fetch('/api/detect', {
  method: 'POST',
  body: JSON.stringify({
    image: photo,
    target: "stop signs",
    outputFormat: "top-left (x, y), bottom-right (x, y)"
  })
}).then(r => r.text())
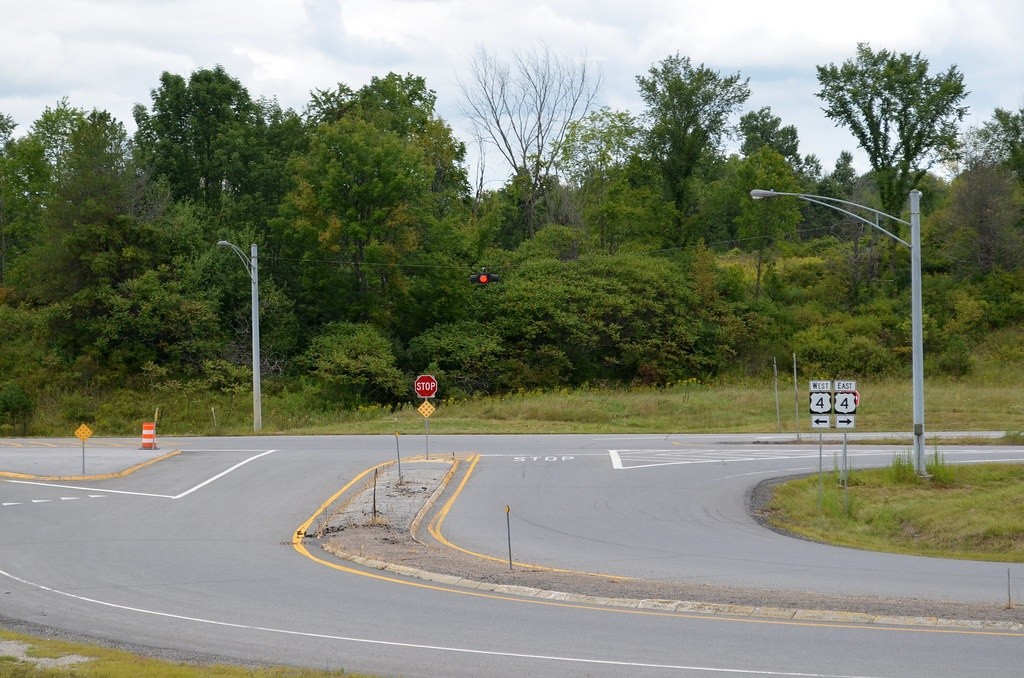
top-left (414, 375), bottom-right (437, 398)
top-left (840, 390), bottom-right (860, 407)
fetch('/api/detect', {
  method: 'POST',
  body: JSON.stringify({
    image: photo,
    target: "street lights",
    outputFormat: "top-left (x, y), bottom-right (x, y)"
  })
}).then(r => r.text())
top-left (218, 241), bottom-right (261, 436)
top-left (749, 189), bottom-right (926, 479)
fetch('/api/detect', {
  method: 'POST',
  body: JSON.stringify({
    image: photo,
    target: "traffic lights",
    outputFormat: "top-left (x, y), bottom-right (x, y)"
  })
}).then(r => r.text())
top-left (471, 272), bottom-right (500, 284)
